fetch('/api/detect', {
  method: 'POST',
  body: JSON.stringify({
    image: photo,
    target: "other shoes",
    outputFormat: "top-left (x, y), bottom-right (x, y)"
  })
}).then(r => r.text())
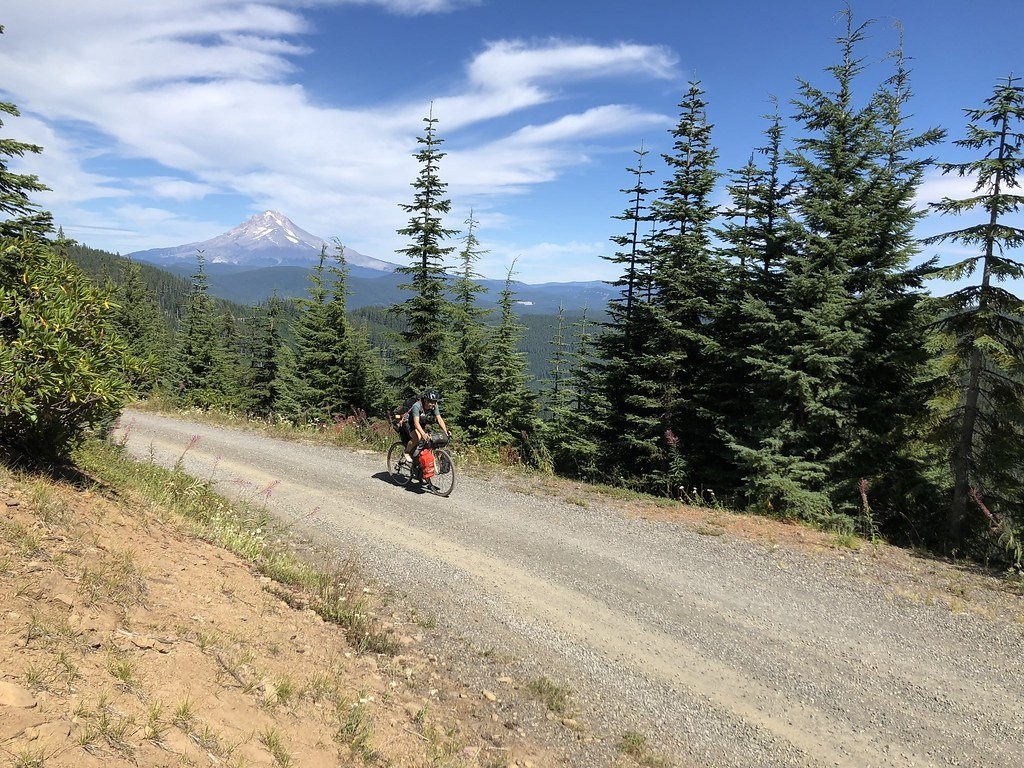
top-left (402, 450), bottom-right (413, 463)
top-left (412, 473), bottom-right (428, 484)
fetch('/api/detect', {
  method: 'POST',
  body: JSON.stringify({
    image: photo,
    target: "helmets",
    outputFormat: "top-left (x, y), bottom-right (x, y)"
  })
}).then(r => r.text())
top-left (424, 389), bottom-right (439, 401)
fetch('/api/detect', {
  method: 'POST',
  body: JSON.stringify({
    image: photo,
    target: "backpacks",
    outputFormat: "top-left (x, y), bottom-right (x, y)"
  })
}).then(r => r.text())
top-left (392, 394), bottom-right (423, 433)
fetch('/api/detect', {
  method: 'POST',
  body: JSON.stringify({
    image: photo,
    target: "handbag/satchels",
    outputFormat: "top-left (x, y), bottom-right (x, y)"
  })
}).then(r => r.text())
top-left (438, 449), bottom-right (450, 473)
top-left (419, 449), bottom-right (435, 479)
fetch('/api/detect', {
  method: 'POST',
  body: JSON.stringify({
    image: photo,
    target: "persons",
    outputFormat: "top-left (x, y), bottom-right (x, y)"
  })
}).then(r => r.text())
top-left (399, 389), bottom-right (451, 485)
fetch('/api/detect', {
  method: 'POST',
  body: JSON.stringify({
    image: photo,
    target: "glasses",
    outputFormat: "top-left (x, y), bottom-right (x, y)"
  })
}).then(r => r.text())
top-left (427, 399), bottom-right (436, 404)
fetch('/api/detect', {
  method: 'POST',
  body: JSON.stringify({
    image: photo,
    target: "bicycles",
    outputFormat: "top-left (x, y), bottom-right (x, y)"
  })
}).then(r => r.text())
top-left (386, 425), bottom-right (456, 498)
